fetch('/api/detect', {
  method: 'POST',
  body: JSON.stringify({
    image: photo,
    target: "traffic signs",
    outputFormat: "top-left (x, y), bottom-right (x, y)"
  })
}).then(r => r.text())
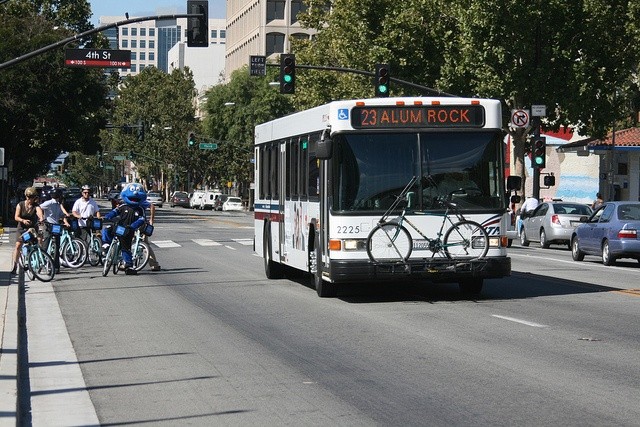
top-left (511, 109), bottom-right (529, 127)
top-left (250, 55), bottom-right (267, 75)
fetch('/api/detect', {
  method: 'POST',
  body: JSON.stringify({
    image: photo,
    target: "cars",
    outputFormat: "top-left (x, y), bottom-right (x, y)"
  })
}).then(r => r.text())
top-left (222, 196), bottom-right (244, 211)
top-left (64, 189), bottom-right (92, 212)
top-left (214, 194), bottom-right (235, 210)
top-left (520, 202), bottom-right (599, 248)
top-left (571, 201), bottom-right (639, 265)
top-left (145, 192), bottom-right (163, 207)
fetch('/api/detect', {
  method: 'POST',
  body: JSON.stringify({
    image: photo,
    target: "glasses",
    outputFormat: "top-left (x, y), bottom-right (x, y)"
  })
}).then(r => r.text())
top-left (27, 195), bottom-right (35, 198)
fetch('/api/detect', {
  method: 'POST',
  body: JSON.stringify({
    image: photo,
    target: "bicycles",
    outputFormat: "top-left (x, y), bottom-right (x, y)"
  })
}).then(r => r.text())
top-left (130, 218), bottom-right (154, 271)
top-left (41, 224), bottom-right (88, 268)
top-left (65, 216), bottom-right (102, 265)
top-left (18, 221), bottom-right (54, 281)
top-left (366, 192), bottom-right (489, 268)
top-left (102, 218), bottom-right (137, 276)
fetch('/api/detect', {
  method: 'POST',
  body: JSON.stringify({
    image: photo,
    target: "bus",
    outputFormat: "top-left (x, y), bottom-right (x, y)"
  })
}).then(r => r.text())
top-left (253, 96), bottom-right (510, 297)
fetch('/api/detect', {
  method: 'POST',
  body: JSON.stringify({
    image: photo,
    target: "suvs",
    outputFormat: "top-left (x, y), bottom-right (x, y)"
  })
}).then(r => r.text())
top-left (188, 191), bottom-right (206, 208)
top-left (171, 193), bottom-right (188, 208)
top-left (200, 191), bottom-right (222, 209)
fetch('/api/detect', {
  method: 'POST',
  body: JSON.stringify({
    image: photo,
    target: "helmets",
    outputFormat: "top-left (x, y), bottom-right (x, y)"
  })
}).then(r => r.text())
top-left (51, 189), bottom-right (62, 198)
top-left (82, 184), bottom-right (90, 189)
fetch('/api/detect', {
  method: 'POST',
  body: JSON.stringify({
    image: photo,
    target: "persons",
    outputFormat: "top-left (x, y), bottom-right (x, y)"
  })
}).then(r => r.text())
top-left (63, 217), bottom-right (70, 227)
top-left (10, 186), bottom-right (43, 275)
top-left (591, 192), bottom-right (604, 210)
top-left (39, 187), bottom-right (63, 274)
top-left (521, 195), bottom-right (539, 215)
top-left (143, 200), bottom-right (161, 272)
top-left (72, 184), bottom-right (103, 254)
top-left (111, 198), bottom-right (123, 210)
top-left (597, 199), bottom-right (604, 209)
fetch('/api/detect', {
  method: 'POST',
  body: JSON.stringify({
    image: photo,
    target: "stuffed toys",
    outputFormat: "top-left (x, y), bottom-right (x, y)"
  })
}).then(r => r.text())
top-left (99, 182), bottom-right (147, 275)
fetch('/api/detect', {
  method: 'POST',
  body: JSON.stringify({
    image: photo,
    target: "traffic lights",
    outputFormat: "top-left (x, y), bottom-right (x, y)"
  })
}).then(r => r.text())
top-left (137, 120), bottom-right (144, 141)
top-left (127, 123), bottom-right (132, 134)
top-left (187, 0), bottom-right (209, 47)
top-left (544, 175), bottom-right (555, 186)
top-left (129, 150), bottom-right (133, 159)
top-left (531, 137), bottom-right (545, 167)
top-left (121, 123), bottom-right (127, 133)
top-left (375, 64), bottom-right (389, 97)
top-left (280, 54), bottom-right (295, 93)
top-left (188, 132), bottom-right (195, 150)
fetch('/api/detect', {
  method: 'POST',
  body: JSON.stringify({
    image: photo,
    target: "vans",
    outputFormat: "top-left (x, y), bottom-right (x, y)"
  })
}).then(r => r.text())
top-left (171, 190), bottom-right (188, 201)
